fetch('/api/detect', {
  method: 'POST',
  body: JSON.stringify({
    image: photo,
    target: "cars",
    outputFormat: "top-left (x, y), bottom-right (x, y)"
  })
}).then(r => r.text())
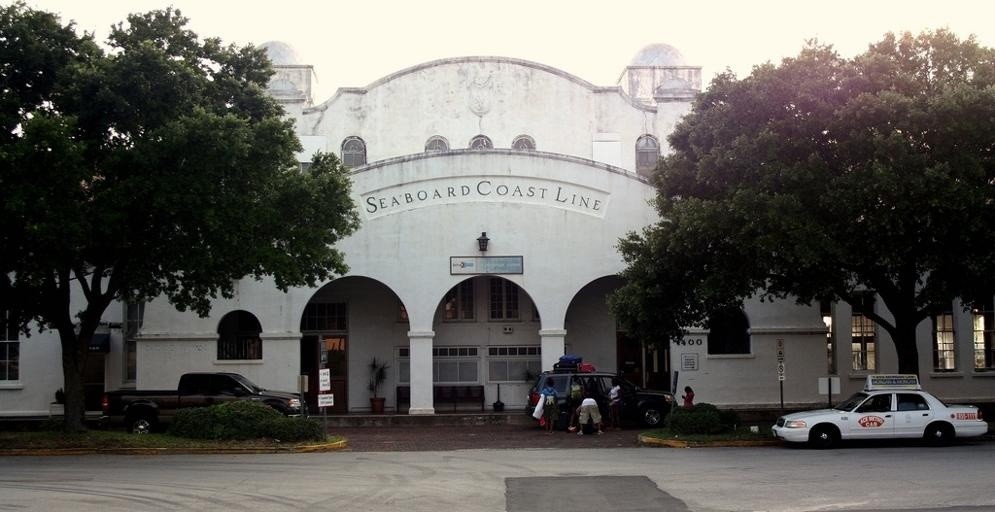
top-left (770, 374), bottom-right (989, 446)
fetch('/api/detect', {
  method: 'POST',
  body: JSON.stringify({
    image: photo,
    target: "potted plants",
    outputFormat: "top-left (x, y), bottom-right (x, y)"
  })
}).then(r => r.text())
top-left (367, 356), bottom-right (391, 412)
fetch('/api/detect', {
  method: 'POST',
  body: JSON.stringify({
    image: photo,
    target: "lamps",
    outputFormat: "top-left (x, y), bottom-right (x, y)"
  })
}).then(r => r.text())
top-left (476, 232), bottom-right (490, 251)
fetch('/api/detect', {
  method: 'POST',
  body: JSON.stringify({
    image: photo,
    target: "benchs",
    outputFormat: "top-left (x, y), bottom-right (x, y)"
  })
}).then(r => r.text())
top-left (396, 385), bottom-right (485, 413)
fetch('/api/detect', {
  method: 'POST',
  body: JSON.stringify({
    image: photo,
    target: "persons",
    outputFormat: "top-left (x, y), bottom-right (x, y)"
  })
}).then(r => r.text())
top-left (539, 377), bottom-right (561, 438)
top-left (569, 375), bottom-right (583, 427)
top-left (576, 397), bottom-right (605, 436)
top-left (682, 385), bottom-right (695, 410)
top-left (607, 378), bottom-right (623, 432)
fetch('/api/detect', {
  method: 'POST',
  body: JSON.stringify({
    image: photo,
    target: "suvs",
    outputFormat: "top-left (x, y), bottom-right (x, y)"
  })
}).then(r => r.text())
top-left (524, 363), bottom-right (680, 429)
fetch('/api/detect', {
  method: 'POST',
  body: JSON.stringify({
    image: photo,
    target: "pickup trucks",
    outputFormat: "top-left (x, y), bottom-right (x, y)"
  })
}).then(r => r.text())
top-left (99, 370), bottom-right (310, 436)
